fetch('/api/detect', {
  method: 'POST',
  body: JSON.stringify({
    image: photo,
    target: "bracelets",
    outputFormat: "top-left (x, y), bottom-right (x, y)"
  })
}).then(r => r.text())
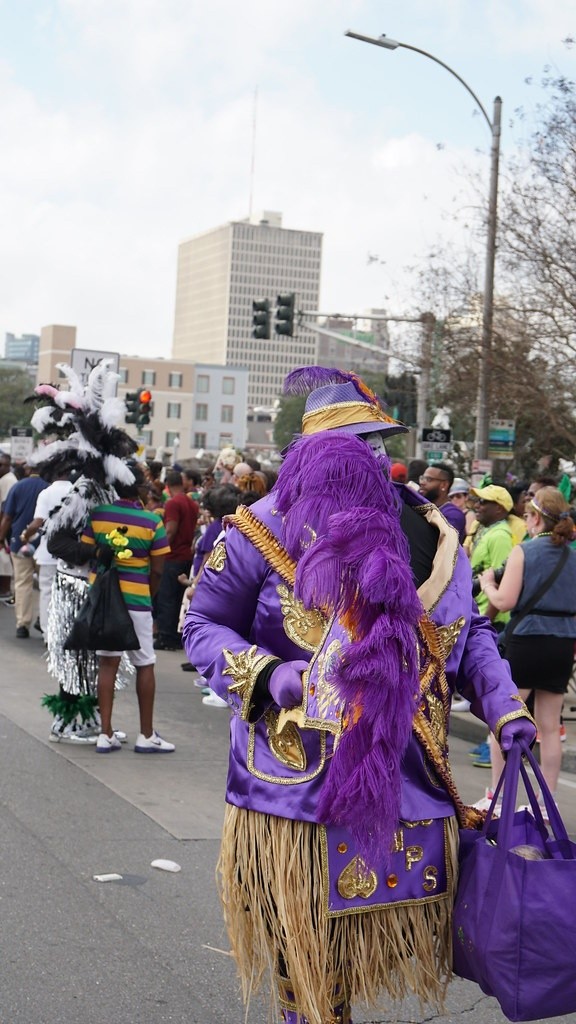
top-left (21, 534), bottom-right (26, 541)
top-left (482, 584), bottom-right (492, 593)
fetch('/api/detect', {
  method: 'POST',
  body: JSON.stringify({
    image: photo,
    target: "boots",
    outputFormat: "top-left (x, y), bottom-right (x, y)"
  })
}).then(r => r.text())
top-left (277, 972), bottom-right (353, 1024)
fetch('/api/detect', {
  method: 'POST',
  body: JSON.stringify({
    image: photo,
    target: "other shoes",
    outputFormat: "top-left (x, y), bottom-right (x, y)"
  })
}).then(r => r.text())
top-left (42, 694), bottom-right (98, 745)
top-left (16, 626), bottom-right (29, 637)
top-left (33, 616), bottom-right (43, 633)
top-left (153, 632), bottom-right (228, 707)
top-left (80, 697), bottom-right (127, 741)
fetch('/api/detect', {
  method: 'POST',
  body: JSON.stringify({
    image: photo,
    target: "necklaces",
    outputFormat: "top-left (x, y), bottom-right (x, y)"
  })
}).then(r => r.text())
top-left (537, 532), bottom-right (553, 537)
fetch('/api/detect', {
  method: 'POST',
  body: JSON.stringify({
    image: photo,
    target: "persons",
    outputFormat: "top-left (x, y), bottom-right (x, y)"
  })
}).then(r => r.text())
top-left (391, 455), bottom-right (570, 714)
top-left (44, 451), bottom-right (127, 744)
top-left (472, 487), bottom-right (576, 823)
top-left (0, 454), bottom-right (74, 645)
top-left (182, 366), bottom-right (539, 1024)
top-left (147, 449), bottom-right (278, 709)
top-left (91, 462), bottom-right (175, 753)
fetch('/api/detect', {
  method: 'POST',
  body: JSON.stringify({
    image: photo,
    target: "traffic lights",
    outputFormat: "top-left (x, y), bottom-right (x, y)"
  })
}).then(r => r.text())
top-left (381, 373), bottom-right (417, 428)
top-left (135, 388), bottom-right (152, 425)
top-left (273, 292), bottom-right (296, 337)
top-left (251, 296), bottom-right (272, 340)
top-left (124, 391), bottom-right (137, 424)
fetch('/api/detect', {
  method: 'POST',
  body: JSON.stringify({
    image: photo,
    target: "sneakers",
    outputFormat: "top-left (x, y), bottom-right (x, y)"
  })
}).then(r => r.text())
top-left (97, 728), bottom-right (122, 753)
top-left (450, 693), bottom-right (566, 822)
top-left (134, 729), bottom-right (175, 753)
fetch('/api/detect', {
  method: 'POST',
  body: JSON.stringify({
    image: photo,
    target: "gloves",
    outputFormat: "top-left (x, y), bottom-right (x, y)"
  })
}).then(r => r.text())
top-left (498, 715), bottom-right (537, 762)
top-left (268, 660), bottom-right (309, 710)
top-left (95, 544), bottom-right (115, 569)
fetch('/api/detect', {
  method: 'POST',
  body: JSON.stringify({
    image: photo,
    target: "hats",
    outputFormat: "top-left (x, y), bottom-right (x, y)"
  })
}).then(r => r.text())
top-left (448, 478), bottom-right (471, 497)
top-left (391, 463), bottom-right (406, 480)
top-left (280, 366), bottom-right (409, 460)
top-left (473, 484), bottom-right (514, 512)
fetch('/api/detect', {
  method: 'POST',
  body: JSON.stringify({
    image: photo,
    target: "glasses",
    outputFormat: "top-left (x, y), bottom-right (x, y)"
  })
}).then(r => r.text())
top-left (524, 491), bottom-right (535, 498)
top-left (419, 476), bottom-right (446, 482)
top-left (450, 493), bottom-right (462, 499)
top-left (473, 497), bottom-right (498, 505)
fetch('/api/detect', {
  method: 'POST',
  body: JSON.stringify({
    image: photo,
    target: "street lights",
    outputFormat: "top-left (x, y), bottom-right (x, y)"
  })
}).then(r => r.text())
top-left (171, 437), bottom-right (181, 467)
top-left (343, 27), bottom-right (505, 489)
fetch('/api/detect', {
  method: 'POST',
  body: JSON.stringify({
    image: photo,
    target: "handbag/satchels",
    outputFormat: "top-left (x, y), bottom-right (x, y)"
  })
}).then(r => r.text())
top-left (446, 738), bottom-right (576, 1021)
top-left (62, 559), bottom-right (141, 652)
